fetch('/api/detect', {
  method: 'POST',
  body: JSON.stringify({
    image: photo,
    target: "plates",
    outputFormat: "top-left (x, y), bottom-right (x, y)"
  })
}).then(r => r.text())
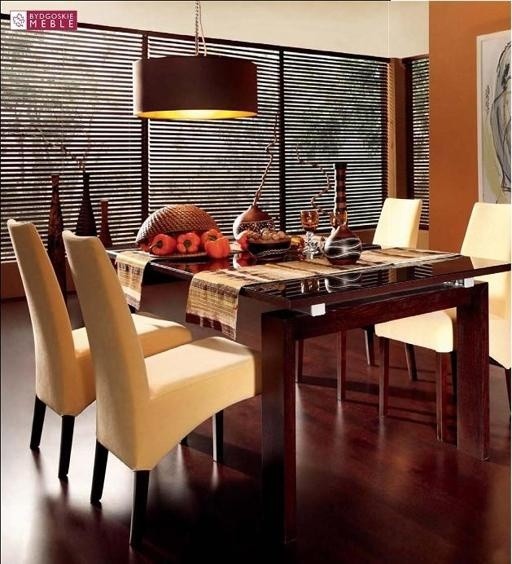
top-left (148, 251), bottom-right (207, 260)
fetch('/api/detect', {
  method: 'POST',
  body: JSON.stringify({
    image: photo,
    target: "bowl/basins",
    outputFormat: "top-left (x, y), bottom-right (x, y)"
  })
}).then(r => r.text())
top-left (246, 237), bottom-right (291, 261)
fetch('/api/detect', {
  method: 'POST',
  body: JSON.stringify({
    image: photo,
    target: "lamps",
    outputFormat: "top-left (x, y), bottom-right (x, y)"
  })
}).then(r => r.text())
top-left (132, 3), bottom-right (259, 122)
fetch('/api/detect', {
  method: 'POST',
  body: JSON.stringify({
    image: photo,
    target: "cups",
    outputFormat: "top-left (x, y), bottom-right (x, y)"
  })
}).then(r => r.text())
top-left (329, 210), bottom-right (348, 230)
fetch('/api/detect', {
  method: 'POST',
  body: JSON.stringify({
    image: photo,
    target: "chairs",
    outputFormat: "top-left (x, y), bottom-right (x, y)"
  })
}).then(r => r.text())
top-left (362, 195), bottom-right (512, 430)
top-left (5, 219), bottom-right (261, 546)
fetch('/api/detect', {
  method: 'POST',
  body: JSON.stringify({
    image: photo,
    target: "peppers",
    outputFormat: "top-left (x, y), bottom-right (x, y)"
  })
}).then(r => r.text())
top-left (205, 236), bottom-right (230, 259)
top-left (177, 232), bottom-right (201, 254)
top-left (149, 234), bottom-right (176, 256)
top-left (200, 229), bottom-right (222, 250)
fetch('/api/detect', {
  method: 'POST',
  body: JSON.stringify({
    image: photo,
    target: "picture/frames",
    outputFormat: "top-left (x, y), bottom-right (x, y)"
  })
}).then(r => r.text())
top-left (475, 28), bottom-right (511, 203)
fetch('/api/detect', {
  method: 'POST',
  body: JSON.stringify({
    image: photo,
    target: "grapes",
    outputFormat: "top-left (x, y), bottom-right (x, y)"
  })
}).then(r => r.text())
top-left (247, 228), bottom-right (287, 243)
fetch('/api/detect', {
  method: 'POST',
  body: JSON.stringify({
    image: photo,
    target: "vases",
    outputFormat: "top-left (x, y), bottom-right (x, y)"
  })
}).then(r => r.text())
top-left (324, 226), bottom-right (362, 266)
top-left (232, 206), bottom-right (274, 244)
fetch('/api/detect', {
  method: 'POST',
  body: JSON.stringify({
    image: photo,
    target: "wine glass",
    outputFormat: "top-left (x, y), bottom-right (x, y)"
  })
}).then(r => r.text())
top-left (300, 210), bottom-right (320, 254)
